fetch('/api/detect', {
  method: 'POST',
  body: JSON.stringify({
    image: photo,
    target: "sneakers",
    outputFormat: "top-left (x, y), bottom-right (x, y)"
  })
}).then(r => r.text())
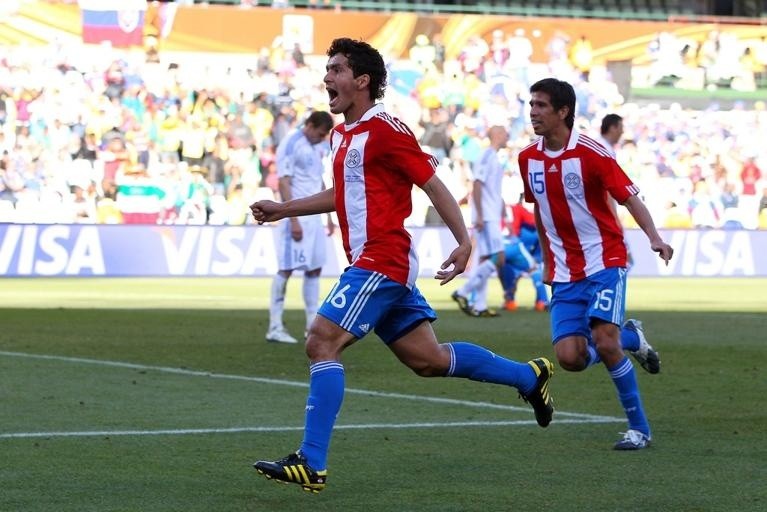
top-left (252, 450), bottom-right (327, 494)
top-left (452, 291), bottom-right (501, 318)
top-left (265, 327), bottom-right (298, 344)
top-left (622, 319), bottom-right (661, 375)
top-left (504, 300), bottom-right (545, 311)
top-left (518, 357), bottom-right (555, 428)
top-left (614, 429), bottom-right (653, 451)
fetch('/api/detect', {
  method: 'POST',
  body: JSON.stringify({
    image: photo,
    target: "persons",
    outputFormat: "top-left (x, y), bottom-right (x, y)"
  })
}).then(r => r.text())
top-left (514, 75), bottom-right (676, 452)
top-left (516, 191), bottom-right (554, 308)
top-left (446, 122), bottom-right (514, 322)
top-left (247, 33), bottom-right (557, 497)
top-left (0, 28), bottom-right (767, 231)
top-left (263, 108), bottom-right (336, 346)
top-left (491, 198), bottom-right (549, 314)
